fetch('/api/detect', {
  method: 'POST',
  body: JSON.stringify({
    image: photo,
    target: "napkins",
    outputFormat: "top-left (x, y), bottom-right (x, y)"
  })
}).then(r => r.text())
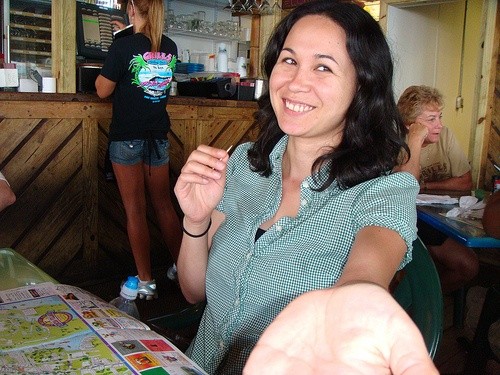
top-left (416, 194), bottom-right (459, 205)
top-left (445, 196), bottom-right (478, 218)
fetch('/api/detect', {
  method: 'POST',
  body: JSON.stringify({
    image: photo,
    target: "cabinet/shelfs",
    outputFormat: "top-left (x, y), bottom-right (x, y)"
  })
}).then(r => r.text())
top-left (0, 0), bottom-right (76, 93)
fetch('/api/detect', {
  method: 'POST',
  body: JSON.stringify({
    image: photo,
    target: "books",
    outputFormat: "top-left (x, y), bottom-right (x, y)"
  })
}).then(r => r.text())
top-left (110, 23), bottom-right (134, 39)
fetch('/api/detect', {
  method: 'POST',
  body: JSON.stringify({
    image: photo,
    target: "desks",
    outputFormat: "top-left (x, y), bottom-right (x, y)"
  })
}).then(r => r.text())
top-left (416, 188), bottom-right (500, 375)
top-left (0, 246), bottom-right (62, 292)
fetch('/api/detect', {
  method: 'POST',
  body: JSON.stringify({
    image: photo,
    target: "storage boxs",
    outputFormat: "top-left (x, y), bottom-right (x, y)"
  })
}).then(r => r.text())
top-left (176, 74), bottom-right (269, 101)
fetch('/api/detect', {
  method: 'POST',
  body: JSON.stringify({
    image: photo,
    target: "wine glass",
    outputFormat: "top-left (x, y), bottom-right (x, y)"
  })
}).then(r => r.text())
top-left (224, 0), bottom-right (286, 15)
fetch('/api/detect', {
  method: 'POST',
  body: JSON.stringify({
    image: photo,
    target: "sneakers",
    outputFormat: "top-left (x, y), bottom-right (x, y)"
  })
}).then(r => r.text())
top-left (119, 275), bottom-right (159, 300)
top-left (166, 263), bottom-right (179, 280)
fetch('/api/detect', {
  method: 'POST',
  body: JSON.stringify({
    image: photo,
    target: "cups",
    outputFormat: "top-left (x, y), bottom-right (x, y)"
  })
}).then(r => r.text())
top-left (236, 56), bottom-right (247, 79)
top-left (218, 43), bottom-right (227, 72)
top-left (163, 9), bottom-right (245, 42)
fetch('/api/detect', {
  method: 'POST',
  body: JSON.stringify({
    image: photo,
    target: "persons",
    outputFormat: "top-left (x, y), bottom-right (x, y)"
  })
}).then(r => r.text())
top-left (173, 0), bottom-right (441, 374)
top-left (457, 189), bottom-right (500, 375)
top-left (389, 86), bottom-right (480, 298)
top-left (0, 171), bottom-right (17, 212)
top-left (94, 0), bottom-right (182, 302)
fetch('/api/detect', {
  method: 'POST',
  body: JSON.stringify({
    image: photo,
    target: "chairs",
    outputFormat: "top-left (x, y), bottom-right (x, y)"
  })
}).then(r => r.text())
top-left (140, 234), bottom-right (445, 362)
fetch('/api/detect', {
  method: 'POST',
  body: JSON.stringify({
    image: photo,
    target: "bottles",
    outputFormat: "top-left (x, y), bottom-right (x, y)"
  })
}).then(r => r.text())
top-left (109, 277), bottom-right (140, 320)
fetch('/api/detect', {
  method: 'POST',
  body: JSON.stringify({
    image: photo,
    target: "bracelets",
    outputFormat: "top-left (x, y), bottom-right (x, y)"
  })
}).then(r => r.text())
top-left (182, 218), bottom-right (213, 238)
top-left (424, 183), bottom-right (427, 192)
